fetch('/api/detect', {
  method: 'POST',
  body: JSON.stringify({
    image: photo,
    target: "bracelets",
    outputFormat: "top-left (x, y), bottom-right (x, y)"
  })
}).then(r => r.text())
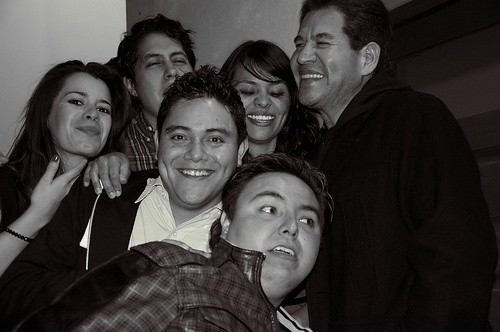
top-left (3, 228), bottom-right (35, 243)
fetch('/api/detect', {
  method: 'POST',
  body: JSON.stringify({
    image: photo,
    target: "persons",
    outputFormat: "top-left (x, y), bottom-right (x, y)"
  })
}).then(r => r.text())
top-left (104, 56), bottom-right (117, 72)
top-left (14, 152), bottom-right (312, 332)
top-left (75, 63), bottom-right (249, 274)
top-left (0, 61), bottom-right (132, 332)
top-left (289, 0), bottom-right (497, 332)
top-left (220, 39), bottom-right (299, 162)
top-left (109, 12), bottom-right (199, 171)
